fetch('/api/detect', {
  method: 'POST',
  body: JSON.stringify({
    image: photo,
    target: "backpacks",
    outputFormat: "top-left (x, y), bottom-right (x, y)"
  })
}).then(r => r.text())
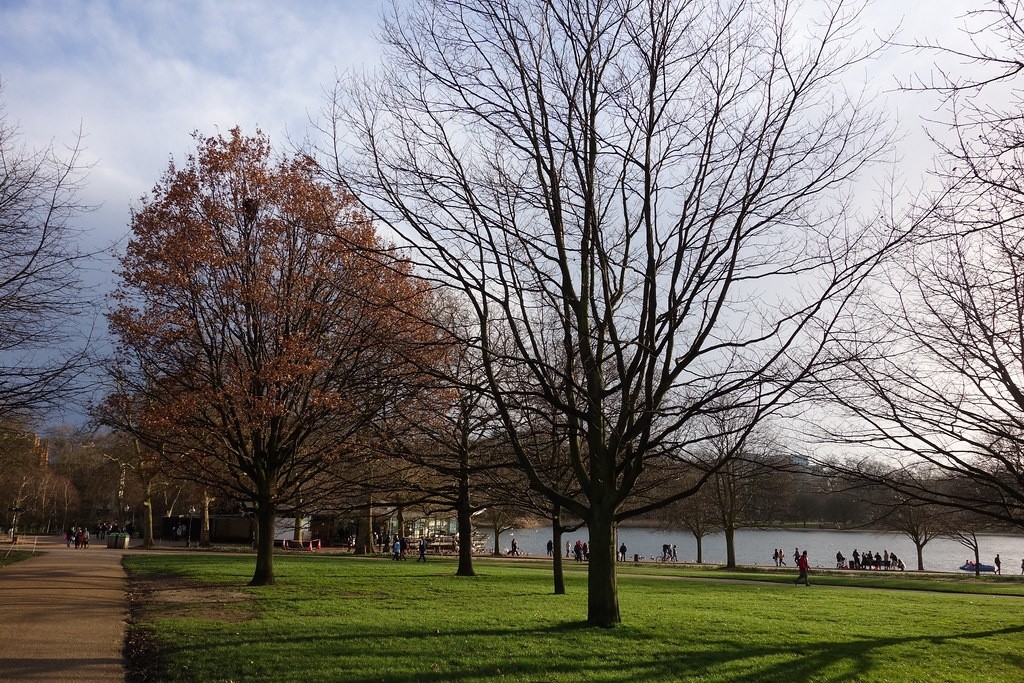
top-left (995, 557), bottom-right (997, 563)
top-left (663, 545), bottom-right (668, 547)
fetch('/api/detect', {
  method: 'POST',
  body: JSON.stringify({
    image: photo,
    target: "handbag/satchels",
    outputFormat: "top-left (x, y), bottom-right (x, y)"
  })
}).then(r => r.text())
top-left (799, 555), bottom-right (800, 557)
top-left (64, 535), bottom-right (68, 541)
top-left (779, 565), bottom-right (781, 566)
top-left (773, 556), bottom-right (775, 559)
top-left (86, 540), bottom-right (89, 544)
top-left (779, 554), bottom-right (784, 558)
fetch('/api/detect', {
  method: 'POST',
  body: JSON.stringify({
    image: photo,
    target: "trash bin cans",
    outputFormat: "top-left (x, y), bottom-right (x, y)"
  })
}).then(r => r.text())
top-left (634, 553), bottom-right (641, 563)
top-left (116, 533), bottom-right (130, 549)
top-left (849, 561), bottom-right (854, 570)
top-left (107, 532), bottom-right (120, 549)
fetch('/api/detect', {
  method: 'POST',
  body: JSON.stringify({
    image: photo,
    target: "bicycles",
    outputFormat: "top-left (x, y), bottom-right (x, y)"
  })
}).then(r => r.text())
top-left (656, 551), bottom-right (676, 564)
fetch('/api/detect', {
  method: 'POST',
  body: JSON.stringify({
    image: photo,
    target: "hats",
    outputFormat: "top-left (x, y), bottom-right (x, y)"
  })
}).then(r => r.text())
top-left (1022, 559), bottom-right (1024, 561)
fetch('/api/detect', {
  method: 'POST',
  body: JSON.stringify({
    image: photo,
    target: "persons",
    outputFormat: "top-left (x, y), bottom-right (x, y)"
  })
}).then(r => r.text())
top-left (346, 531), bottom-right (629, 564)
top-left (965, 554), bottom-right (1024, 577)
top-left (834, 549), bottom-right (907, 573)
top-left (7, 519), bottom-right (190, 549)
top-left (773, 547), bottom-right (802, 566)
top-left (662, 543), bottom-right (677, 562)
top-left (793, 550), bottom-right (812, 589)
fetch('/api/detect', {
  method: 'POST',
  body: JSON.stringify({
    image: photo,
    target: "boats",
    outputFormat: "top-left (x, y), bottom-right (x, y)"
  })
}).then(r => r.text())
top-left (960, 564), bottom-right (996, 572)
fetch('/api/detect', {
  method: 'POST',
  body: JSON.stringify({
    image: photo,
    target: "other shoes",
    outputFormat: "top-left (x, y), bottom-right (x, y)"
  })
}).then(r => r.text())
top-left (806, 584), bottom-right (811, 586)
top-left (794, 580), bottom-right (797, 585)
top-left (777, 565), bottom-right (778, 566)
top-left (785, 564), bottom-right (786, 566)
top-left (994, 571), bottom-right (996, 574)
top-left (796, 565), bottom-right (799, 567)
top-left (999, 574), bottom-right (1001, 575)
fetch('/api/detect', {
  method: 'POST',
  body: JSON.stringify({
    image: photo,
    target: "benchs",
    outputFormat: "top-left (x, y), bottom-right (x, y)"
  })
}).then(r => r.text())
top-left (860, 560), bottom-right (891, 570)
